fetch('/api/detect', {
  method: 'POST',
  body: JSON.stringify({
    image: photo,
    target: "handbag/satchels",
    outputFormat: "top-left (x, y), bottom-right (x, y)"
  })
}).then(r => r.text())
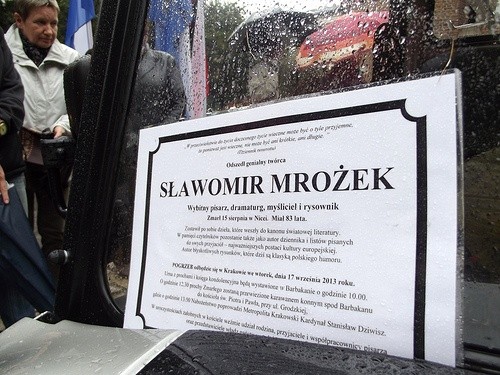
top-left (40, 138), bottom-right (75, 167)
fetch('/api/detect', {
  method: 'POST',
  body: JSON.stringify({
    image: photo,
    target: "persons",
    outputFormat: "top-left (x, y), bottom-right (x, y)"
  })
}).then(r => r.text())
top-left (0, 0), bottom-right (185, 328)
top-left (239, 40), bottom-right (365, 100)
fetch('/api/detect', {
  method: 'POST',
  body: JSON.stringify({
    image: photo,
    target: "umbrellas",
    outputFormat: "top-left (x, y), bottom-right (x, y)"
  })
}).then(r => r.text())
top-left (226, 2), bottom-right (387, 69)
